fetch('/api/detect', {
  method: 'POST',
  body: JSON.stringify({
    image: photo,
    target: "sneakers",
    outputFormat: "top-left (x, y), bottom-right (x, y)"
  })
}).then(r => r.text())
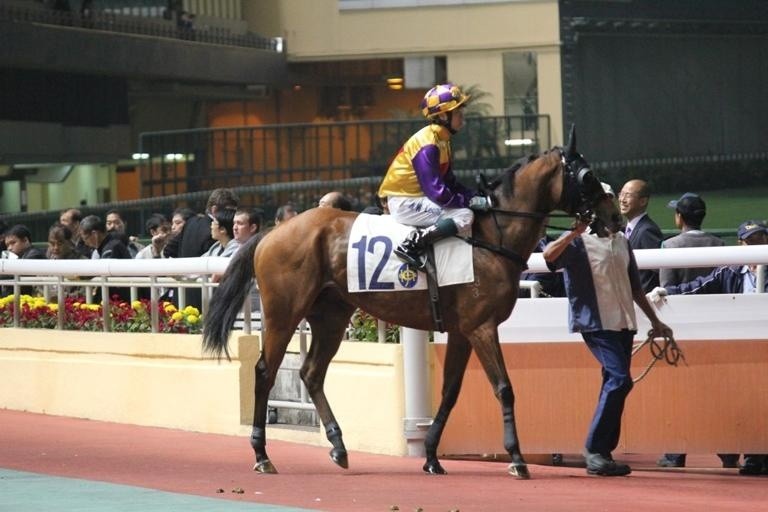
top-left (719, 460), bottom-right (741, 469)
top-left (654, 455), bottom-right (686, 468)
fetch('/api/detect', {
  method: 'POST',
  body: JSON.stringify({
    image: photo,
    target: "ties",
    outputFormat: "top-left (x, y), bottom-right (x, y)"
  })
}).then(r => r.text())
top-left (622, 226), bottom-right (632, 241)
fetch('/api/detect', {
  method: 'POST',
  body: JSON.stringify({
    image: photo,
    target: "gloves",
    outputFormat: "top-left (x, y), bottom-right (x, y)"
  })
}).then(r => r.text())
top-left (646, 286), bottom-right (669, 304)
top-left (467, 194), bottom-right (498, 212)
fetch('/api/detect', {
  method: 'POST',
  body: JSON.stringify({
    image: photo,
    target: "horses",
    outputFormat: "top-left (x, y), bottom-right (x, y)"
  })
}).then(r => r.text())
top-left (202, 122), bottom-right (623, 481)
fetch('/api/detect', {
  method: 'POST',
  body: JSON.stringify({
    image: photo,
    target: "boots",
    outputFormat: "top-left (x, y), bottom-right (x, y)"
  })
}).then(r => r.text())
top-left (391, 224), bottom-right (443, 274)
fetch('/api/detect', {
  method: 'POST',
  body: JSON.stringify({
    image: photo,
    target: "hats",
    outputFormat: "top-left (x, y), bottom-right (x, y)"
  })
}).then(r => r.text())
top-left (737, 219), bottom-right (768, 242)
top-left (600, 181), bottom-right (615, 198)
top-left (665, 193), bottom-right (707, 219)
top-left (420, 80), bottom-right (471, 120)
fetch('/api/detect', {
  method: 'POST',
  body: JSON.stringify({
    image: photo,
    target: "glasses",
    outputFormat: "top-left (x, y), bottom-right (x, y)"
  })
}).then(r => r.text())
top-left (617, 192), bottom-right (640, 201)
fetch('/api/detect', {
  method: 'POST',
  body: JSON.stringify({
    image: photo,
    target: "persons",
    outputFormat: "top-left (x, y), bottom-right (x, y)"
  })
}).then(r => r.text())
top-left (518, 217), bottom-right (565, 297)
top-left (646, 220), bottom-right (768, 474)
top-left (48, 224), bottom-right (77, 259)
top-left (200, 208), bottom-right (239, 282)
top-left (163, 187), bottom-right (240, 312)
top-left (655, 193), bottom-right (740, 468)
top-left (543, 181), bottom-right (664, 476)
top-left (618, 179), bottom-right (662, 294)
top-left (378, 84), bottom-right (488, 273)
top-left (135, 214), bottom-right (172, 298)
top-left (5, 224), bottom-right (46, 259)
top-left (375, 190), bottom-right (390, 214)
top-left (152, 208), bottom-right (196, 302)
top-left (318, 191), bottom-right (352, 212)
top-left (274, 204), bottom-right (297, 225)
top-left (80, 215), bottom-right (132, 258)
top-left (61, 209), bottom-right (89, 256)
top-left (232, 208), bottom-right (260, 258)
top-left (91, 208), bottom-right (136, 258)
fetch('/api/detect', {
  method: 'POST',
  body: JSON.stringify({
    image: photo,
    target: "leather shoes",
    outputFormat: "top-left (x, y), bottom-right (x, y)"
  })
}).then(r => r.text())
top-left (582, 452), bottom-right (615, 476)
top-left (605, 455), bottom-right (631, 477)
